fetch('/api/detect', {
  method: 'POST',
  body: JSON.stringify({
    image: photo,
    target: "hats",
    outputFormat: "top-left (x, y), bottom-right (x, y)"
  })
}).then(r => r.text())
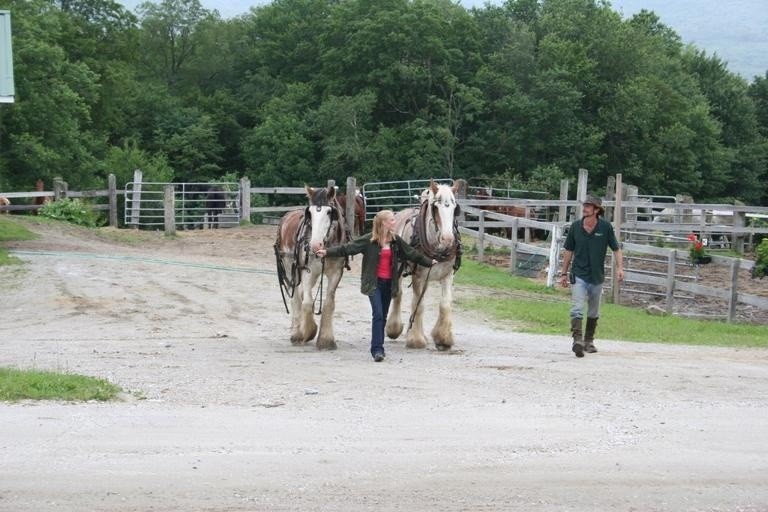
top-left (579, 194), bottom-right (606, 218)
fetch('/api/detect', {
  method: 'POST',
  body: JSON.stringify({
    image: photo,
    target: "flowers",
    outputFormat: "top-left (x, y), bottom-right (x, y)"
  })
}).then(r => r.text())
top-left (684, 235), bottom-right (705, 265)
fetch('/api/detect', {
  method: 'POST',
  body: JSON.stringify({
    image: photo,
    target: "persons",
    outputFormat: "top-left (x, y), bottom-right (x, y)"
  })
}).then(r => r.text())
top-left (318, 210), bottom-right (438, 362)
top-left (560, 194), bottom-right (625, 357)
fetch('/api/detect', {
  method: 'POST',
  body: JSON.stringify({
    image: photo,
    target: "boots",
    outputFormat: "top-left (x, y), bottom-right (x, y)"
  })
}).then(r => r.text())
top-left (583, 316), bottom-right (600, 353)
top-left (571, 317), bottom-right (583, 357)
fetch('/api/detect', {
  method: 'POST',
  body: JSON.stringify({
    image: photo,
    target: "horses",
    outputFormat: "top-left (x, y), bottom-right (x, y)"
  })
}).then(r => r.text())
top-left (190, 180), bottom-right (225, 229)
top-left (274, 181), bottom-right (351, 352)
top-left (473, 184), bottom-right (537, 244)
top-left (0, 193), bottom-right (14, 215)
top-left (338, 193), bottom-right (366, 236)
top-left (384, 176), bottom-right (468, 351)
top-left (31, 176), bottom-right (51, 214)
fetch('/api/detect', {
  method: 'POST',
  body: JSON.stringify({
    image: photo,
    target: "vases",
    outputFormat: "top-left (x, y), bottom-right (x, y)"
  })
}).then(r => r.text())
top-left (693, 255), bottom-right (712, 265)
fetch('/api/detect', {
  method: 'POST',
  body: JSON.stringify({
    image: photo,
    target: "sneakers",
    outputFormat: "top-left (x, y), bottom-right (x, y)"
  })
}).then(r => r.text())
top-left (373, 352), bottom-right (385, 361)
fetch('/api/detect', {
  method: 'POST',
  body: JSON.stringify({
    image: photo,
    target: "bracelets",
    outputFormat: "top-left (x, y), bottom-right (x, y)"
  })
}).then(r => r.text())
top-left (562, 273), bottom-right (568, 276)
